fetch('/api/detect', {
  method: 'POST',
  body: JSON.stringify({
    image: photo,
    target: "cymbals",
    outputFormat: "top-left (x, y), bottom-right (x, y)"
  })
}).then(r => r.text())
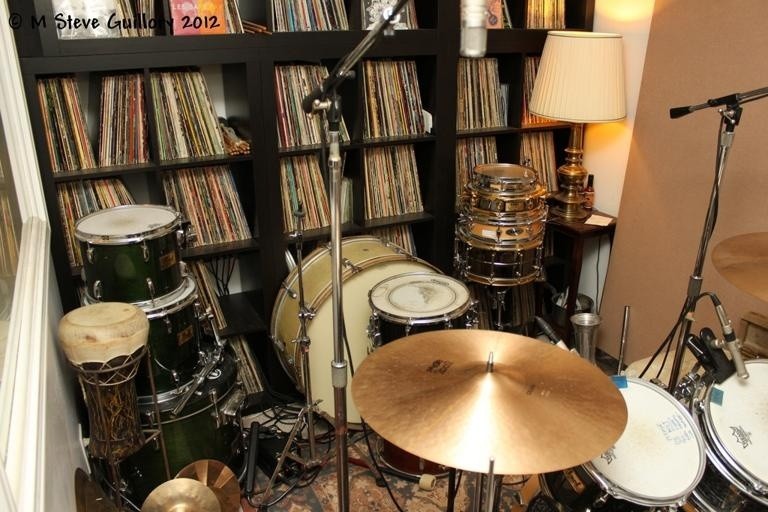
top-left (140, 478), bottom-right (222, 512)
top-left (352, 330), bottom-right (628, 477)
top-left (712, 231), bottom-right (768, 303)
top-left (75, 467), bottom-right (119, 512)
top-left (173, 460), bottom-right (242, 512)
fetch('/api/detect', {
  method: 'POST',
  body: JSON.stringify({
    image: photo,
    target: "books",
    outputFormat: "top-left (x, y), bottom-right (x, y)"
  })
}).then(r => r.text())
top-left (489, 1), bottom-right (567, 32)
top-left (114, 0), bottom-right (354, 38)
top-left (34, 60), bottom-right (566, 395)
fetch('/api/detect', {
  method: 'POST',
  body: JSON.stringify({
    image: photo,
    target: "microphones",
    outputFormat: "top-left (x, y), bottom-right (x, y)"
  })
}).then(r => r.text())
top-left (704, 292), bottom-right (750, 382)
top-left (457, 0), bottom-right (490, 61)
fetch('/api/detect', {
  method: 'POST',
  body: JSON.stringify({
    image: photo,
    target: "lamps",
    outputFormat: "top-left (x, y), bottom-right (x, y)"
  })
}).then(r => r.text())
top-left (527, 26), bottom-right (627, 222)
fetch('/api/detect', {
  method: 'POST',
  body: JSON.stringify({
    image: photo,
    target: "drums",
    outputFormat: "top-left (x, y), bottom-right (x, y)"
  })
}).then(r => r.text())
top-left (691, 358), bottom-right (767, 511)
top-left (364, 272), bottom-right (481, 481)
top-left (92, 348), bottom-right (249, 512)
top-left (73, 205), bottom-right (199, 309)
top-left (539, 376), bottom-right (708, 509)
top-left (267, 234), bottom-right (446, 432)
top-left (452, 163), bottom-right (549, 288)
top-left (58, 301), bottom-right (151, 463)
top-left (80, 275), bottom-right (212, 404)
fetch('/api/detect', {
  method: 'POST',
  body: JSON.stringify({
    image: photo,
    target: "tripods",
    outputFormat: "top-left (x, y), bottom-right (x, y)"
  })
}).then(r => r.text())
top-left (256, 232), bottom-right (386, 512)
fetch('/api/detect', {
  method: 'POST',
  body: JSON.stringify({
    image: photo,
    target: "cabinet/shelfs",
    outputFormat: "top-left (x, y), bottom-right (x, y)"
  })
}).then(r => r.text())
top-left (11, 0), bottom-right (596, 417)
